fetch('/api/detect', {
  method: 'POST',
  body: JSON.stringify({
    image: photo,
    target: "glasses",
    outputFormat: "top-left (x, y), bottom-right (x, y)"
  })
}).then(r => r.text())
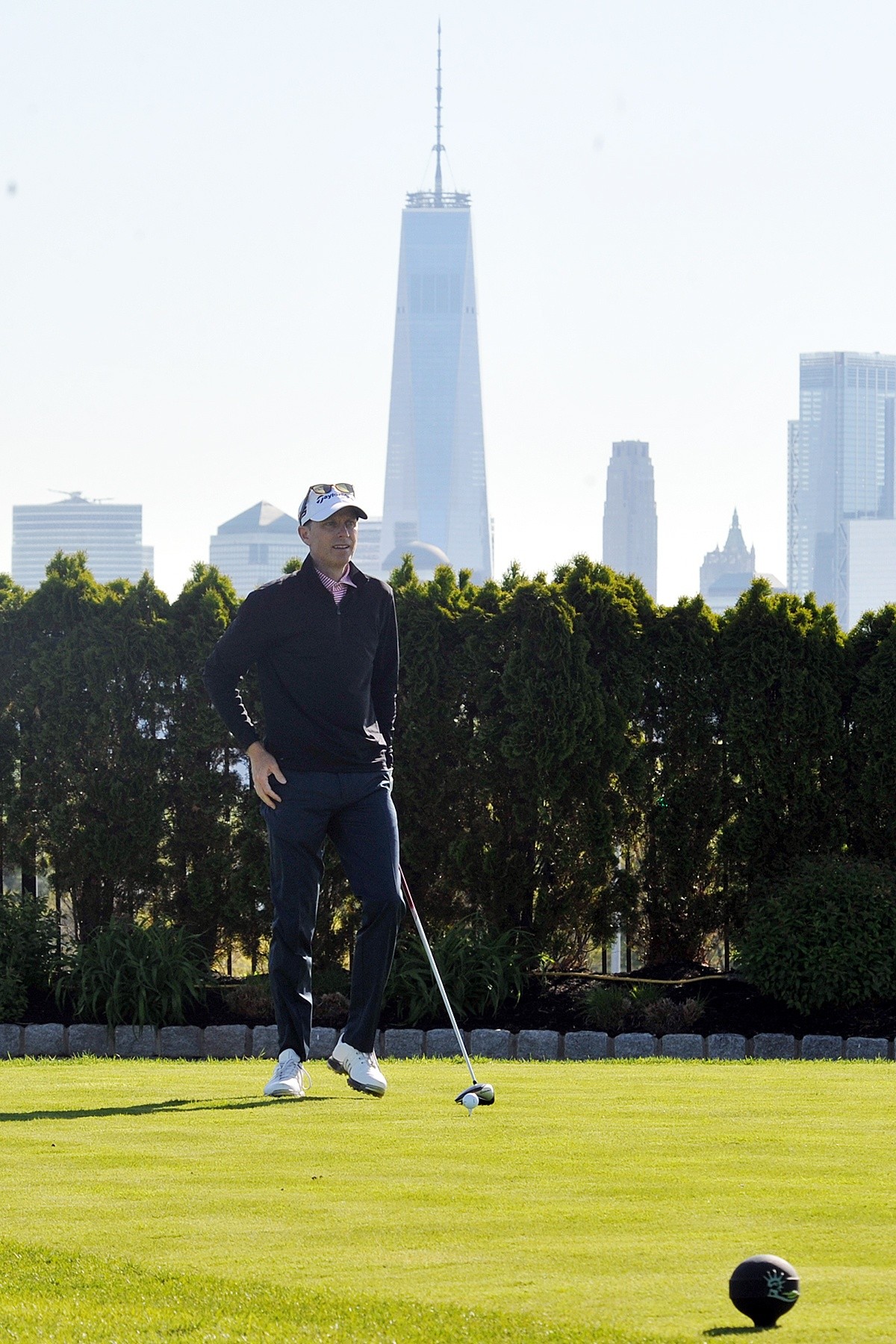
top-left (299, 483), bottom-right (355, 526)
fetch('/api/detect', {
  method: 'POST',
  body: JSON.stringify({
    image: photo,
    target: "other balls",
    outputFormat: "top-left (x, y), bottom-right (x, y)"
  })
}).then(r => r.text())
top-left (728, 1253), bottom-right (802, 1320)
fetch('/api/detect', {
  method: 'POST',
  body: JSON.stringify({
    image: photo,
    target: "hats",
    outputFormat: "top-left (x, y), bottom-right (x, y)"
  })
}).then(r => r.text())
top-left (298, 486), bottom-right (368, 526)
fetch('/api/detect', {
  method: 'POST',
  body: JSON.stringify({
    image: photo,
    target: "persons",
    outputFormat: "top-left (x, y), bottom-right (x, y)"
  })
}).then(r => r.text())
top-left (206, 483), bottom-right (406, 1098)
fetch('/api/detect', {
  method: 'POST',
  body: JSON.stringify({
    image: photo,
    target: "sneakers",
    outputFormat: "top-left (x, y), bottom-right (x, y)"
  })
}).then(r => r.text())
top-left (263, 1048), bottom-right (311, 1097)
top-left (327, 1033), bottom-right (387, 1099)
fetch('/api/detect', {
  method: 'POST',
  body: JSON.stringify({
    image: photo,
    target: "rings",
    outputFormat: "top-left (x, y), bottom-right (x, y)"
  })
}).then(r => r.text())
top-left (253, 784), bottom-right (256, 789)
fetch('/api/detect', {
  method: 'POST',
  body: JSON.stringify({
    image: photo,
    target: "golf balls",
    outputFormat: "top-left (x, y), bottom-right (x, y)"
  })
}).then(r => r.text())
top-left (463, 1092), bottom-right (479, 1109)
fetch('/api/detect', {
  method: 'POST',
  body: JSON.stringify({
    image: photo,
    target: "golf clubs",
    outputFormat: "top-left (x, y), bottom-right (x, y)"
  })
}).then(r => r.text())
top-left (399, 863), bottom-right (498, 1106)
